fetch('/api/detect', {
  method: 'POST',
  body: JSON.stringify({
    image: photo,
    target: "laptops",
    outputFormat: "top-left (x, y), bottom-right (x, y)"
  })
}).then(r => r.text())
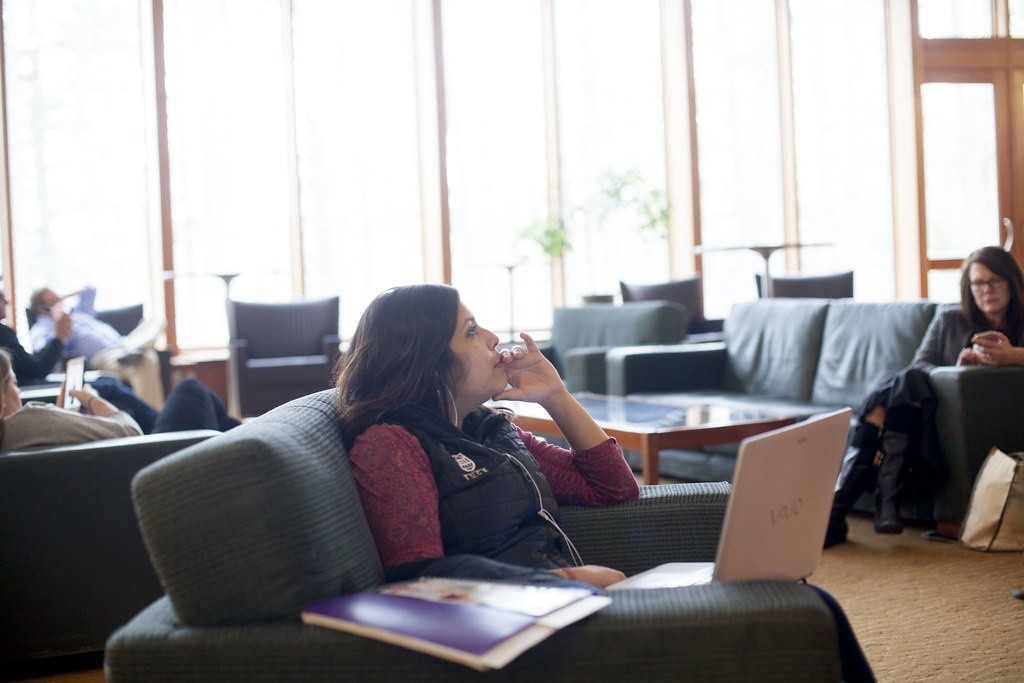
top-left (604, 407), bottom-right (854, 594)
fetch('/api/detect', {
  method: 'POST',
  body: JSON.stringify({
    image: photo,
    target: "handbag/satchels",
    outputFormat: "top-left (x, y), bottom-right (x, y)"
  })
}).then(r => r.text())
top-left (957, 445), bottom-right (1024, 552)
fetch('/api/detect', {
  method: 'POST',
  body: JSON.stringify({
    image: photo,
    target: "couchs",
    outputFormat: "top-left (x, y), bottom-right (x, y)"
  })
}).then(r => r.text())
top-left (226, 296), bottom-right (342, 418)
top-left (583, 276), bottom-right (726, 343)
top-left (538, 304), bottom-right (686, 393)
top-left (0, 306), bottom-right (224, 662)
top-left (100, 387), bottom-right (846, 683)
top-left (755, 271), bottom-right (855, 298)
top-left (605, 296), bottom-right (1024, 540)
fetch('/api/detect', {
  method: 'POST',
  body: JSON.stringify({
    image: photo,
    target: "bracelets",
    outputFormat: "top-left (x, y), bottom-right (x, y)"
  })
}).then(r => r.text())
top-left (561, 568), bottom-right (575, 579)
top-left (87, 395), bottom-right (97, 414)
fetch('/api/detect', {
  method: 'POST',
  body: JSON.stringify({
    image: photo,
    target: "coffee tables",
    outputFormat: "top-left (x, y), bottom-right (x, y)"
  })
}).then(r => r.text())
top-left (479, 390), bottom-right (795, 485)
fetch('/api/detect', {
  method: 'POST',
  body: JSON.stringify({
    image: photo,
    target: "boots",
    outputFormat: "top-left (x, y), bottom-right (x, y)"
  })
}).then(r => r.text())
top-left (873, 431), bottom-right (908, 536)
top-left (822, 446), bottom-right (871, 550)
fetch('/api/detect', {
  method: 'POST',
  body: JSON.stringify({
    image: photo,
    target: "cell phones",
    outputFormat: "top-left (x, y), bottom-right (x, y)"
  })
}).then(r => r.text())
top-left (973, 331), bottom-right (999, 343)
top-left (63, 356), bottom-right (86, 413)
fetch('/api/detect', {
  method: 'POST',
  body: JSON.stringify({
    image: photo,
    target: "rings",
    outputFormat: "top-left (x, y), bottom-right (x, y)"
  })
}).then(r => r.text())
top-left (984, 353), bottom-right (991, 361)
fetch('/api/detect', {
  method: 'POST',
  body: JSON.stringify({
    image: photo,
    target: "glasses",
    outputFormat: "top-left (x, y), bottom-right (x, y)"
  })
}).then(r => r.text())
top-left (969, 277), bottom-right (1005, 292)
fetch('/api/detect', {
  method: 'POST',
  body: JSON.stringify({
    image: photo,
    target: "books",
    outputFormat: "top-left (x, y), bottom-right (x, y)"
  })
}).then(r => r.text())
top-left (297, 578), bottom-right (613, 671)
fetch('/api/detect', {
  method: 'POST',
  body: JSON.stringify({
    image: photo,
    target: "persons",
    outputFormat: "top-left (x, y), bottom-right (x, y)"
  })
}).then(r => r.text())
top-left (339, 285), bottom-right (640, 588)
top-left (0, 276), bottom-right (71, 378)
top-left (823, 247), bottom-right (1024, 550)
top-left (29, 288), bottom-right (165, 413)
top-left (0, 348), bottom-right (243, 451)
